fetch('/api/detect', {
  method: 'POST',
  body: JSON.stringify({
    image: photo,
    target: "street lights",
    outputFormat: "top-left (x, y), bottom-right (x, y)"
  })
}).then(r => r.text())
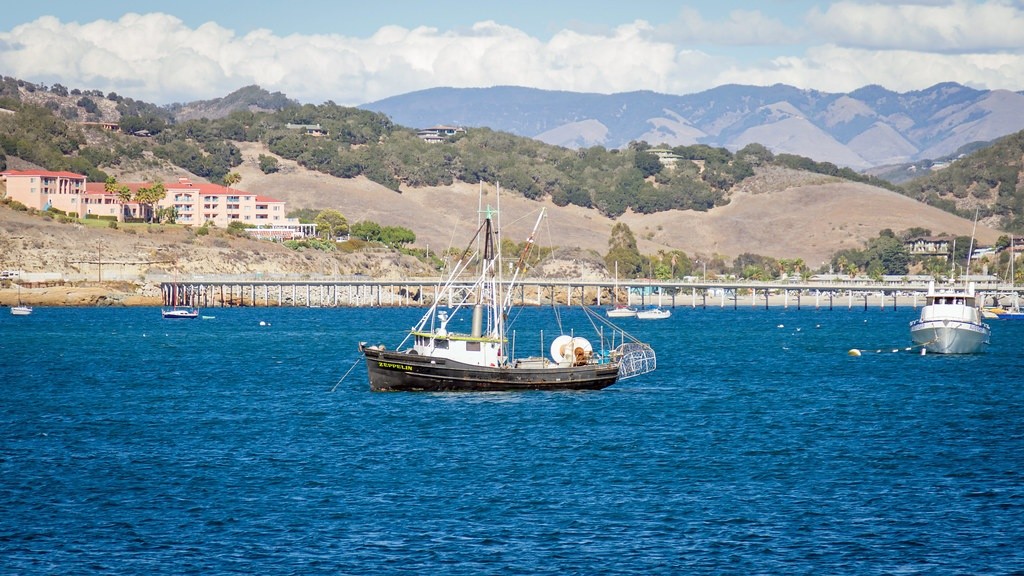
top-left (573, 258), bottom-right (584, 282)
top-left (695, 259), bottom-right (706, 284)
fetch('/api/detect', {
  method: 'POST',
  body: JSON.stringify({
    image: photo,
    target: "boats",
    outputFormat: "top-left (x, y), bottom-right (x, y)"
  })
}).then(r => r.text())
top-left (908, 207), bottom-right (1024, 357)
top-left (356, 175), bottom-right (658, 395)
top-left (10, 249), bottom-right (33, 316)
top-left (161, 303), bottom-right (200, 319)
top-left (636, 308), bottom-right (671, 320)
top-left (606, 260), bottom-right (637, 318)
